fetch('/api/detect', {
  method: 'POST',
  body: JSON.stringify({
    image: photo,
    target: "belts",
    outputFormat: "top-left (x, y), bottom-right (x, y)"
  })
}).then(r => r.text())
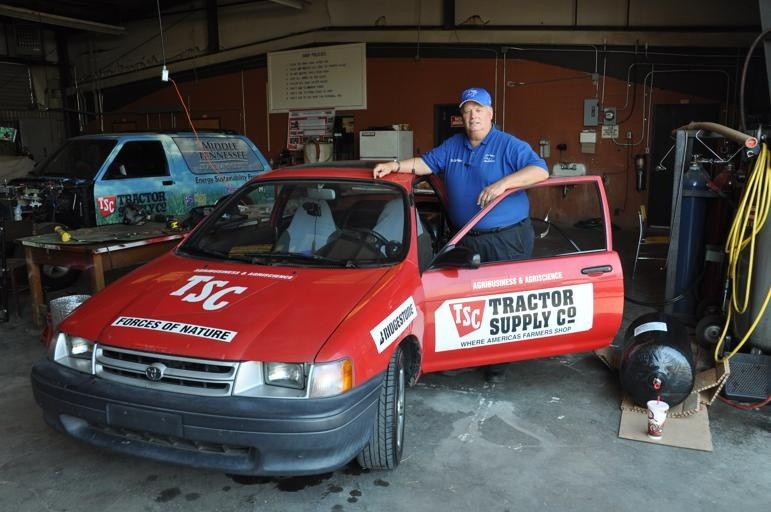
top-left (467, 217), bottom-right (531, 235)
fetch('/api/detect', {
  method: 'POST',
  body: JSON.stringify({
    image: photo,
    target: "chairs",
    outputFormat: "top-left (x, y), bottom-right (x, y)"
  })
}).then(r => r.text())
top-left (632, 205), bottom-right (669, 281)
top-left (0, 220), bottom-right (49, 319)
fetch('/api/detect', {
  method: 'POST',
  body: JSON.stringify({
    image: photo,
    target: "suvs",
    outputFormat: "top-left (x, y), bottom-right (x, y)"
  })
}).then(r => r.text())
top-left (0, 128), bottom-right (282, 290)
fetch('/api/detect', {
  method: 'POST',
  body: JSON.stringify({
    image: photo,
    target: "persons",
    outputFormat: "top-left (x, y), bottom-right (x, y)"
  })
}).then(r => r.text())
top-left (373, 87), bottom-right (549, 383)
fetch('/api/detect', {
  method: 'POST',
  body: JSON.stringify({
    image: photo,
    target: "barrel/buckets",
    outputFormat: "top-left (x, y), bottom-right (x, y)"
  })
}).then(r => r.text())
top-left (303, 140), bottom-right (335, 164)
top-left (622, 311), bottom-right (696, 409)
top-left (49, 294), bottom-right (94, 333)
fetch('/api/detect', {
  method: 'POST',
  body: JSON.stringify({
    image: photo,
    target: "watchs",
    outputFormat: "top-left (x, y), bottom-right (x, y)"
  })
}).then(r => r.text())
top-left (393, 159), bottom-right (401, 173)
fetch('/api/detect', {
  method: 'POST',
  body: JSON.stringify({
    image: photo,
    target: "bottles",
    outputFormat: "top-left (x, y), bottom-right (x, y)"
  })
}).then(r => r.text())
top-left (14, 205), bottom-right (23, 221)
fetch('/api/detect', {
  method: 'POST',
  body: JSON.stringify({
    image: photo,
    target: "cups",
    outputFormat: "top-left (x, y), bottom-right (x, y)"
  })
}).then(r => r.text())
top-left (647, 400), bottom-right (670, 440)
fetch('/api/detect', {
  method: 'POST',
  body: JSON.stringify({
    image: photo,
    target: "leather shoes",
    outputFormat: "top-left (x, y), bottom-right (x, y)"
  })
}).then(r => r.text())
top-left (489, 364), bottom-right (508, 383)
top-left (442, 369), bottom-right (465, 377)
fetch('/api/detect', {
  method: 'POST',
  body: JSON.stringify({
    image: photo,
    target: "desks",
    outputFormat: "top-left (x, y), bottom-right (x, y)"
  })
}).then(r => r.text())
top-left (17, 214), bottom-right (271, 331)
top-left (0, 195), bottom-right (57, 294)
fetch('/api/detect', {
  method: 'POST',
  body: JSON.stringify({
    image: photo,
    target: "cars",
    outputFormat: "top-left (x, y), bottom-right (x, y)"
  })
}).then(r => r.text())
top-left (26, 156), bottom-right (626, 482)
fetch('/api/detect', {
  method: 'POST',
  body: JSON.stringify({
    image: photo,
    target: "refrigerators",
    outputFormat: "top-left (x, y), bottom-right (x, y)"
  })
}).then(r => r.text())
top-left (358, 130), bottom-right (415, 161)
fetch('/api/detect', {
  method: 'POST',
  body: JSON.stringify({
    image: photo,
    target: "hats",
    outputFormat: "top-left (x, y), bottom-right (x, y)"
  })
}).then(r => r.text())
top-left (458, 86), bottom-right (493, 108)
